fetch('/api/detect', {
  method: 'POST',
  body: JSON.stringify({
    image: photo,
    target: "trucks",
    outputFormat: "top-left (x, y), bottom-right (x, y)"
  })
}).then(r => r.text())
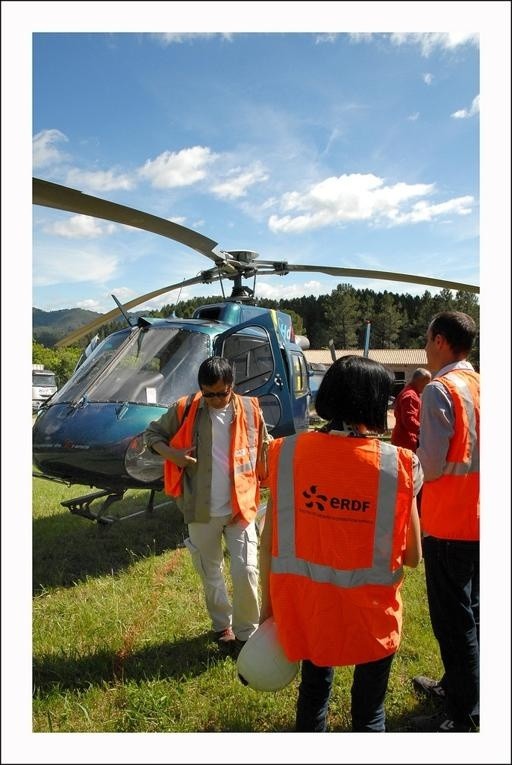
top-left (31, 364), bottom-right (60, 414)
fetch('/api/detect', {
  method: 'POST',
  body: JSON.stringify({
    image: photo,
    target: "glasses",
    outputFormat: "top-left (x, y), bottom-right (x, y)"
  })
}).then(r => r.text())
top-left (201, 386), bottom-right (230, 397)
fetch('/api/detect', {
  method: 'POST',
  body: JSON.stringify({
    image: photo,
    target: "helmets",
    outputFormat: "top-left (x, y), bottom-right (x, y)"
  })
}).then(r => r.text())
top-left (236, 616), bottom-right (300, 693)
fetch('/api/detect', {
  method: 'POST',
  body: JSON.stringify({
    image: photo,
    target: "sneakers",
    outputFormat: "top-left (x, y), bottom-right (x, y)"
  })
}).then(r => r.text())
top-left (414, 676), bottom-right (448, 699)
top-left (218, 625), bottom-right (237, 654)
top-left (411, 709), bottom-right (472, 731)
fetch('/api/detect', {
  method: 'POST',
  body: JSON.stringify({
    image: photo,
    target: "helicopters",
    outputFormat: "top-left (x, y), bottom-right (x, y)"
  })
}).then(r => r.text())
top-left (32, 178), bottom-right (482, 524)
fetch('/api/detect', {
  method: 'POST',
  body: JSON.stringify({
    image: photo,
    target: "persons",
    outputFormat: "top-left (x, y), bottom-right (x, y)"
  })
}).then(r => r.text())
top-left (257, 355), bottom-right (426, 733)
top-left (390, 366), bottom-right (431, 452)
top-left (404, 310), bottom-right (478, 733)
top-left (144, 355), bottom-right (270, 653)
